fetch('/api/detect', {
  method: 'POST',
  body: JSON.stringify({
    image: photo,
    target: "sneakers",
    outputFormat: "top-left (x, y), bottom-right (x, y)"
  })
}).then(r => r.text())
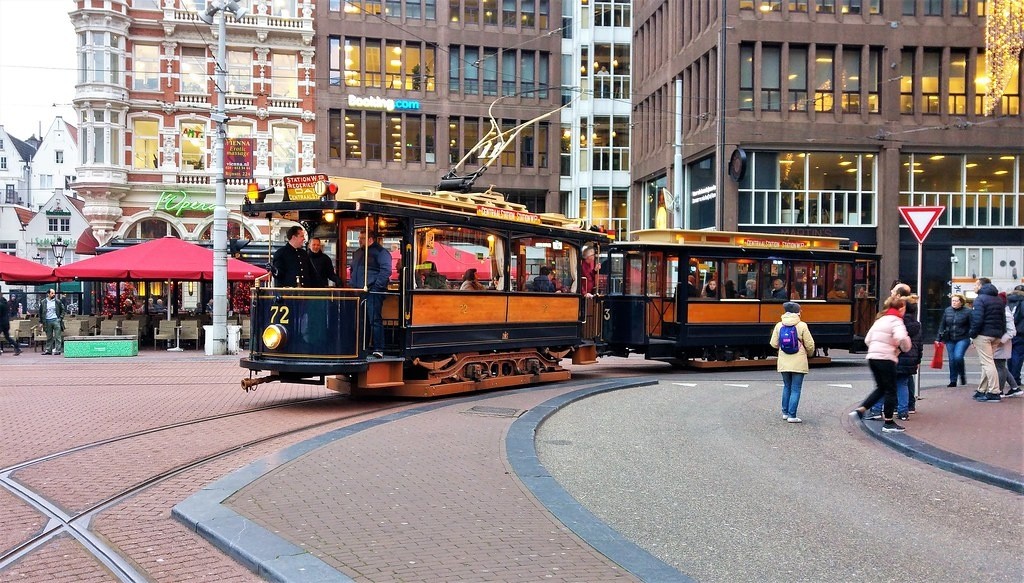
top-left (865, 410), bottom-right (882, 419)
top-left (898, 412), bottom-right (909, 420)
top-left (882, 421), bottom-right (905, 432)
top-left (1005, 388), bottom-right (1023, 397)
top-left (977, 393), bottom-right (1001, 402)
top-left (855, 408), bottom-right (865, 422)
top-left (999, 391), bottom-right (1003, 397)
top-left (973, 390), bottom-right (985, 398)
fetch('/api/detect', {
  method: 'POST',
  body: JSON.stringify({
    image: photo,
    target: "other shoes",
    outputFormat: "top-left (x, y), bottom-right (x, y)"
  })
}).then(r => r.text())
top-left (783, 415), bottom-right (788, 419)
top-left (14, 349), bottom-right (22, 356)
top-left (55, 352), bottom-right (60, 355)
top-left (373, 349), bottom-right (383, 359)
top-left (961, 375), bottom-right (965, 385)
top-left (788, 417), bottom-right (802, 422)
top-left (893, 408), bottom-right (898, 414)
top-left (41, 351), bottom-right (52, 355)
top-left (948, 382), bottom-right (956, 387)
top-left (909, 406), bottom-right (915, 413)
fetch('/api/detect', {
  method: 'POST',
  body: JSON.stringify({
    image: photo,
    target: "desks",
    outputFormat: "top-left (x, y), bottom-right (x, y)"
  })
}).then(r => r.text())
top-left (162, 324), bottom-right (191, 352)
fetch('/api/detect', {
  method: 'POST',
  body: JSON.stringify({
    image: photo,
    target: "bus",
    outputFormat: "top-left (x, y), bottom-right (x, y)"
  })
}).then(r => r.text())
top-left (237, 85), bottom-right (883, 398)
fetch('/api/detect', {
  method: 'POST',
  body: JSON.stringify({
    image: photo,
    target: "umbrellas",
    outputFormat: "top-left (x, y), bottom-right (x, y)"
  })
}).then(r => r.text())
top-left (0, 252), bottom-right (60, 286)
top-left (54, 234), bottom-right (269, 352)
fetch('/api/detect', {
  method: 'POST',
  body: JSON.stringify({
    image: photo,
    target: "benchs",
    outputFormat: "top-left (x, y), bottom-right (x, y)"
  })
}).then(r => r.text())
top-left (62, 335), bottom-right (139, 359)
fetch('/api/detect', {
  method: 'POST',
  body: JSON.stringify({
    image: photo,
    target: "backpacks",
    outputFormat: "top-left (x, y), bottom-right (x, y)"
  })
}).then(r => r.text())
top-left (779, 324), bottom-right (801, 354)
top-left (1008, 300), bottom-right (1022, 324)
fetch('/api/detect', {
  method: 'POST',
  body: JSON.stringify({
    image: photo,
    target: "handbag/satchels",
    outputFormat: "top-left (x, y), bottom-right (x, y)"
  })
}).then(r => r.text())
top-left (932, 341), bottom-right (944, 368)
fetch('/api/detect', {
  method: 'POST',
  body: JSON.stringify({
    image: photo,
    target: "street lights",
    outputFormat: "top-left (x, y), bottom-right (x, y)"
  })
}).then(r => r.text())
top-left (196, 0), bottom-right (249, 354)
top-left (50, 236), bottom-right (69, 300)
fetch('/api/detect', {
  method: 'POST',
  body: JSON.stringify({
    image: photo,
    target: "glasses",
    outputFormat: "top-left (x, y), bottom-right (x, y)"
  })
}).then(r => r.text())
top-left (358, 237), bottom-right (365, 240)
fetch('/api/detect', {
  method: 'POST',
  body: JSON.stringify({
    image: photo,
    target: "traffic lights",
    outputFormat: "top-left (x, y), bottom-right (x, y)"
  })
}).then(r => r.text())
top-left (229, 238), bottom-right (250, 254)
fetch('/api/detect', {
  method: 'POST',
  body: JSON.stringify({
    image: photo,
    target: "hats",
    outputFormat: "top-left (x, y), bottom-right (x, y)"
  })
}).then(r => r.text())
top-left (998, 292), bottom-right (1007, 304)
top-left (783, 302), bottom-right (800, 313)
top-left (1015, 285), bottom-right (1024, 291)
top-left (11, 294), bottom-right (16, 297)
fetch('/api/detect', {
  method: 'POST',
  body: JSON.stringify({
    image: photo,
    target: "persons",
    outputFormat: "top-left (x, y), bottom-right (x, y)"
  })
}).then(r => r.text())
top-left (863, 279), bottom-right (923, 421)
top-left (195, 298), bottom-right (213, 323)
top-left (527, 266), bottom-right (563, 293)
top-left (273, 226), bottom-right (344, 287)
top-left (701, 279), bottom-right (725, 299)
top-left (936, 294), bottom-right (973, 387)
top-left (798, 273), bottom-right (865, 299)
top-left (688, 275), bottom-right (700, 297)
top-left (736, 279), bottom-right (756, 299)
top-left (771, 280), bottom-right (800, 300)
top-left (970, 277), bottom-right (1024, 402)
top-left (724, 280), bottom-right (736, 299)
top-left (122, 297), bottom-right (169, 313)
top-left (415, 260), bottom-right (497, 291)
top-left (848, 296), bottom-right (911, 432)
top-left (770, 302), bottom-right (814, 422)
top-left (352, 228), bottom-right (392, 359)
top-left (0, 285), bottom-right (24, 356)
top-left (39, 288), bottom-right (65, 355)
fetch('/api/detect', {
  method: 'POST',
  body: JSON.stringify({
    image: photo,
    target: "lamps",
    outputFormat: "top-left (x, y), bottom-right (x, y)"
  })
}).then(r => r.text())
top-left (188, 281), bottom-right (194, 296)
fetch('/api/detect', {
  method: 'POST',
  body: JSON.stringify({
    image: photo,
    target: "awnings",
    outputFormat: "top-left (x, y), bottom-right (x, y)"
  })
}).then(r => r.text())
top-left (74, 226), bottom-right (100, 255)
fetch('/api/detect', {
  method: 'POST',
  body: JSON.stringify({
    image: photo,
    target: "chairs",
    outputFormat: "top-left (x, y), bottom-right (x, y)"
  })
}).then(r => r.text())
top-left (227, 319), bottom-right (250, 350)
top-left (178, 319), bottom-right (201, 351)
top-left (62, 312), bottom-right (148, 340)
top-left (153, 318), bottom-right (177, 351)
top-left (8, 318), bottom-right (47, 353)
